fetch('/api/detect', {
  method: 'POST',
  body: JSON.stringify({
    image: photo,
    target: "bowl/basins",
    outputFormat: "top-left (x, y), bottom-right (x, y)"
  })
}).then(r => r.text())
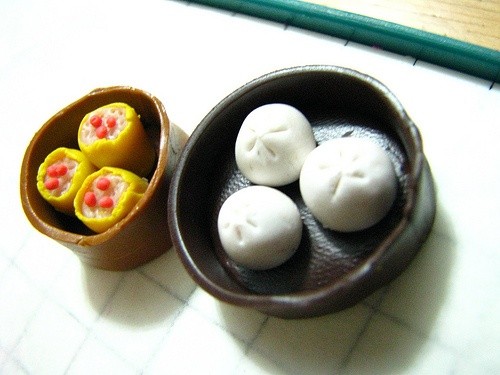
top-left (166, 64), bottom-right (437, 319)
top-left (19, 85), bottom-right (190, 271)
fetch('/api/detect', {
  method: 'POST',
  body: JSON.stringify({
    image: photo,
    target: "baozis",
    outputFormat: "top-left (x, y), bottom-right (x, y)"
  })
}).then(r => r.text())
top-left (299, 137), bottom-right (398, 232)
top-left (235, 103), bottom-right (317, 187)
top-left (217, 185), bottom-right (302, 272)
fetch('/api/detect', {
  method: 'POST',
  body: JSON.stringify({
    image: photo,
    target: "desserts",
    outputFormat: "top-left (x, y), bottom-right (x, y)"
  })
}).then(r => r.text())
top-left (73, 166), bottom-right (149, 234)
top-left (78, 102), bottom-right (155, 174)
top-left (36, 148), bottom-right (96, 213)
